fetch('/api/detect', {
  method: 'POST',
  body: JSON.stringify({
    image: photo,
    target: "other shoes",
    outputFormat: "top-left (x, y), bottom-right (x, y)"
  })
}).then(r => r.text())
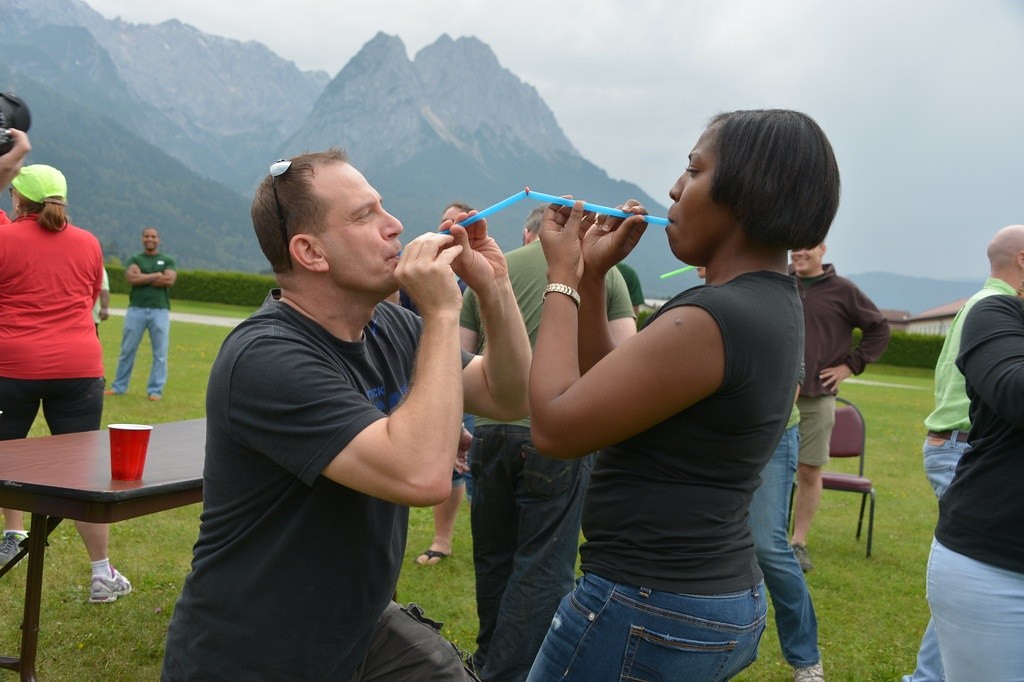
top-left (792, 541), bottom-right (808, 572)
top-left (149, 394), bottom-right (160, 401)
top-left (103, 390), bottom-right (116, 395)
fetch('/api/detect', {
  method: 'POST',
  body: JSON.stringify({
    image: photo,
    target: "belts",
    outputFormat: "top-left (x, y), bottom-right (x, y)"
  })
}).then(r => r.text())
top-left (928, 429), bottom-right (969, 442)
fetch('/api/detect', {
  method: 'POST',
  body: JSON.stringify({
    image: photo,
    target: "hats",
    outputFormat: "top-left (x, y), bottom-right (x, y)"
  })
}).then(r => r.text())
top-left (12, 164), bottom-right (67, 202)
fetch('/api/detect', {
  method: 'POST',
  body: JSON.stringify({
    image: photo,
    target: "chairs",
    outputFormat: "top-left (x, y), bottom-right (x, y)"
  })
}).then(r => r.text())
top-left (0, 417), bottom-right (206, 682)
top-left (786, 397), bottom-right (875, 559)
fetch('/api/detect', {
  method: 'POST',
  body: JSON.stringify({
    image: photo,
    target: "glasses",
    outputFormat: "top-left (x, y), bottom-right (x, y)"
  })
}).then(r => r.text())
top-left (270, 158), bottom-right (292, 270)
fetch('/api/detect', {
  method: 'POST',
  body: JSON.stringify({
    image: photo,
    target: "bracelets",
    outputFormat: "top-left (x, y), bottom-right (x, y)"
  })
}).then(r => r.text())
top-left (461, 423), bottom-right (464, 432)
top-left (542, 283), bottom-right (581, 309)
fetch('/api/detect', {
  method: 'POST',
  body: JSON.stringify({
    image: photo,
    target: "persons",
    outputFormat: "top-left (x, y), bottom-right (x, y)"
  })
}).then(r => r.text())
top-left (617, 262), bottom-right (645, 315)
top-left (105, 228), bottom-right (177, 401)
top-left (92, 268), bottom-right (110, 337)
top-left (904, 297), bottom-right (1024, 682)
top-left (459, 204), bottom-right (636, 682)
top-left (388, 204), bottom-right (475, 566)
top-left (922, 224), bottom-right (1024, 497)
top-left (0, 164), bottom-right (131, 604)
top-left (162, 154), bottom-right (532, 682)
top-left (526, 108), bottom-right (841, 682)
top-left (789, 243), bottom-right (890, 574)
top-left (695, 265), bottom-right (823, 681)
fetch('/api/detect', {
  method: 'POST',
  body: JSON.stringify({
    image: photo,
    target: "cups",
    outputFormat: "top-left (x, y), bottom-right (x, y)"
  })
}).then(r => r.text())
top-left (107, 424), bottom-right (154, 482)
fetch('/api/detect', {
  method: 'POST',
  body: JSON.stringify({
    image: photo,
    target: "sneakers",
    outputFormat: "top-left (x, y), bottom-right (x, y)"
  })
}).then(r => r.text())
top-left (0, 531), bottom-right (27, 564)
top-left (89, 565), bottom-right (132, 603)
top-left (795, 659), bottom-right (823, 682)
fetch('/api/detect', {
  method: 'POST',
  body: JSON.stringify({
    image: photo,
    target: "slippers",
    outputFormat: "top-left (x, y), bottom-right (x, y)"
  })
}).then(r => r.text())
top-left (417, 549), bottom-right (447, 566)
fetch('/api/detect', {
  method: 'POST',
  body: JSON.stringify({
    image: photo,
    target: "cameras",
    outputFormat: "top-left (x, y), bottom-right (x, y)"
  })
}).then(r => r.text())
top-left (0, 92), bottom-right (32, 156)
